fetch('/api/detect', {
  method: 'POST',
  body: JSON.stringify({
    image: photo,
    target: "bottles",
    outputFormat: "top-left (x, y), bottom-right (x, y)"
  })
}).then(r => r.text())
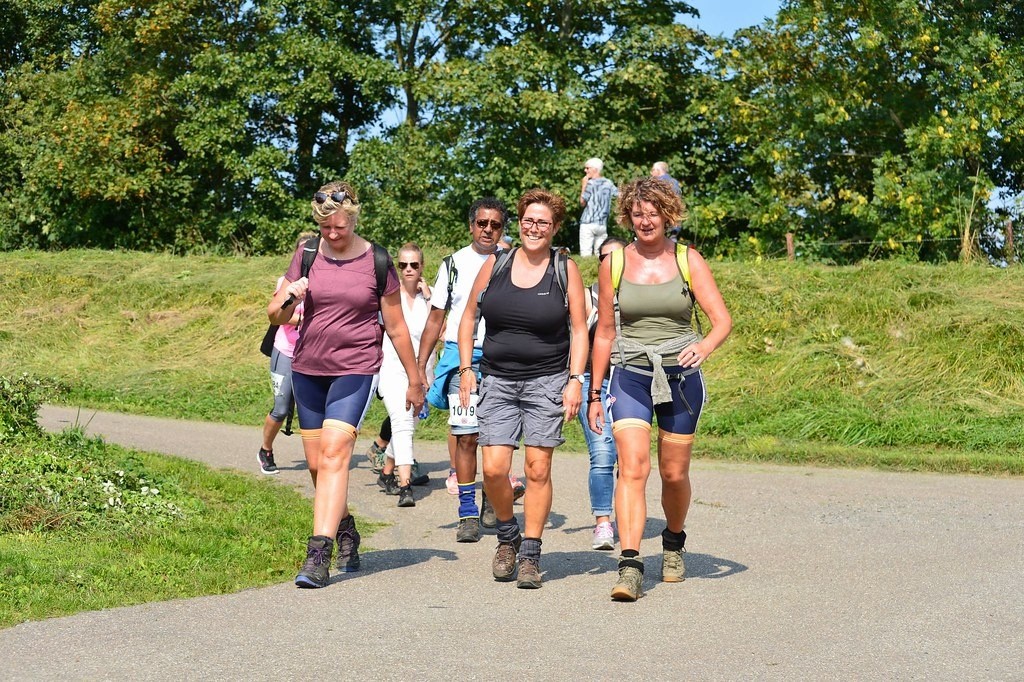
top-left (560, 247), bottom-right (570, 255)
top-left (418, 386), bottom-right (429, 419)
top-left (689, 244), bottom-right (695, 249)
top-left (273, 292), bottom-right (277, 296)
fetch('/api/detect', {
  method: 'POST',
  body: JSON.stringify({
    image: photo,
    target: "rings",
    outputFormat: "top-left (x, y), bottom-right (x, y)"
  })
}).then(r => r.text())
top-left (691, 351), bottom-right (695, 357)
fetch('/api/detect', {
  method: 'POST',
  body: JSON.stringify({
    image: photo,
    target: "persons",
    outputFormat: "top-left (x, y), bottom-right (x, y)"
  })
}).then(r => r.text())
top-left (649, 161), bottom-right (683, 241)
top-left (418, 188), bottom-right (590, 590)
top-left (570, 177), bottom-right (732, 600)
top-left (579, 158), bottom-right (623, 258)
top-left (257, 181), bottom-right (447, 589)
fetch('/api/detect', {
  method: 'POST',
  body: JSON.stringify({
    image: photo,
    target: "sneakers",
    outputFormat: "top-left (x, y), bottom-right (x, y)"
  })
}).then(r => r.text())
top-left (481, 489), bottom-right (496, 527)
top-left (661, 547), bottom-right (684, 582)
top-left (257, 447), bottom-right (279, 474)
top-left (456, 518), bottom-right (479, 542)
top-left (518, 557), bottom-right (541, 588)
top-left (510, 476), bottom-right (525, 501)
top-left (611, 555), bottom-right (643, 598)
top-left (492, 535), bottom-right (521, 579)
top-left (366, 445), bottom-right (385, 474)
top-left (410, 459), bottom-right (429, 485)
top-left (377, 469), bottom-right (401, 495)
top-left (445, 472), bottom-right (459, 494)
top-left (398, 485), bottom-right (415, 507)
top-left (592, 522), bottom-right (615, 550)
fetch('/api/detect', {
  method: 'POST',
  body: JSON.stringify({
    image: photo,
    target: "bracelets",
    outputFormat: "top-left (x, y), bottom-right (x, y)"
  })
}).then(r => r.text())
top-left (588, 388), bottom-right (601, 394)
top-left (458, 367), bottom-right (473, 377)
top-left (587, 397), bottom-right (601, 404)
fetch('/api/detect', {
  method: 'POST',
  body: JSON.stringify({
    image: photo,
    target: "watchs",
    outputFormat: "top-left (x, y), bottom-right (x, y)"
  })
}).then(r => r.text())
top-left (568, 375), bottom-right (585, 384)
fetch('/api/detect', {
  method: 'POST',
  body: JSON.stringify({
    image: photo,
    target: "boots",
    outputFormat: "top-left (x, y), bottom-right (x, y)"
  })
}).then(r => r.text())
top-left (295, 537), bottom-right (334, 588)
top-left (335, 516), bottom-right (360, 573)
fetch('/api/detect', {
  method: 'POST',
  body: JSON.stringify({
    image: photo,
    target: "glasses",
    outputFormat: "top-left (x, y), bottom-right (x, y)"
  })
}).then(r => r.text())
top-left (474, 219), bottom-right (502, 231)
top-left (399, 262), bottom-right (421, 270)
top-left (520, 218), bottom-right (553, 232)
top-left (314, 192), bottom-right (354, 204)
